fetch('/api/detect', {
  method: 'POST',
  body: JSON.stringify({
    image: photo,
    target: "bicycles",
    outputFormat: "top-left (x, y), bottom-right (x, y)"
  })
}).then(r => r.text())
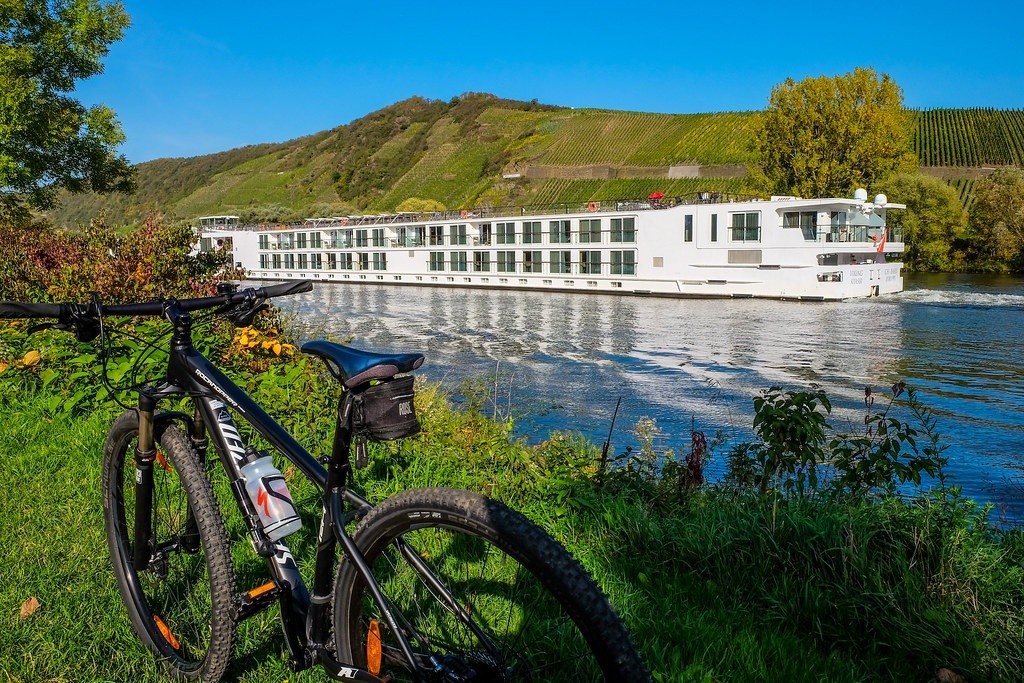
top-left (0, 278), bottom-right (652, 683)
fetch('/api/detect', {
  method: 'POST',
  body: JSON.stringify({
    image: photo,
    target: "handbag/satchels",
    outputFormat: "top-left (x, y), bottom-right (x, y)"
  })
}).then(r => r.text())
top-left (340, 376), bottom-right (421, 469)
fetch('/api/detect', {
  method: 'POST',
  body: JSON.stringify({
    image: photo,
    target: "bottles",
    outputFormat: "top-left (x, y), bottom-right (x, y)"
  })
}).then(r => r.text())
top-left (238, 445), bottom-right (302, 542)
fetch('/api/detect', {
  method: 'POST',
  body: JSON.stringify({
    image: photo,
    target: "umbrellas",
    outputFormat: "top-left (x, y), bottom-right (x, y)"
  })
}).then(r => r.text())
top-left (649, 192), bottom-right (665, 198)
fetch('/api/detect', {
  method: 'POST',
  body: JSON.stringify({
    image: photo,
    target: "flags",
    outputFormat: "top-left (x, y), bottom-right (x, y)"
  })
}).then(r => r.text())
top-left (877, 228), bottom-right (887, 254)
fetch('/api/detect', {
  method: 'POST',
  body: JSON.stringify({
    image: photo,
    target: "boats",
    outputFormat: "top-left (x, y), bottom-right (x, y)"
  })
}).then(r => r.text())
top-left (189, 186), bottom-right (907, 303)
